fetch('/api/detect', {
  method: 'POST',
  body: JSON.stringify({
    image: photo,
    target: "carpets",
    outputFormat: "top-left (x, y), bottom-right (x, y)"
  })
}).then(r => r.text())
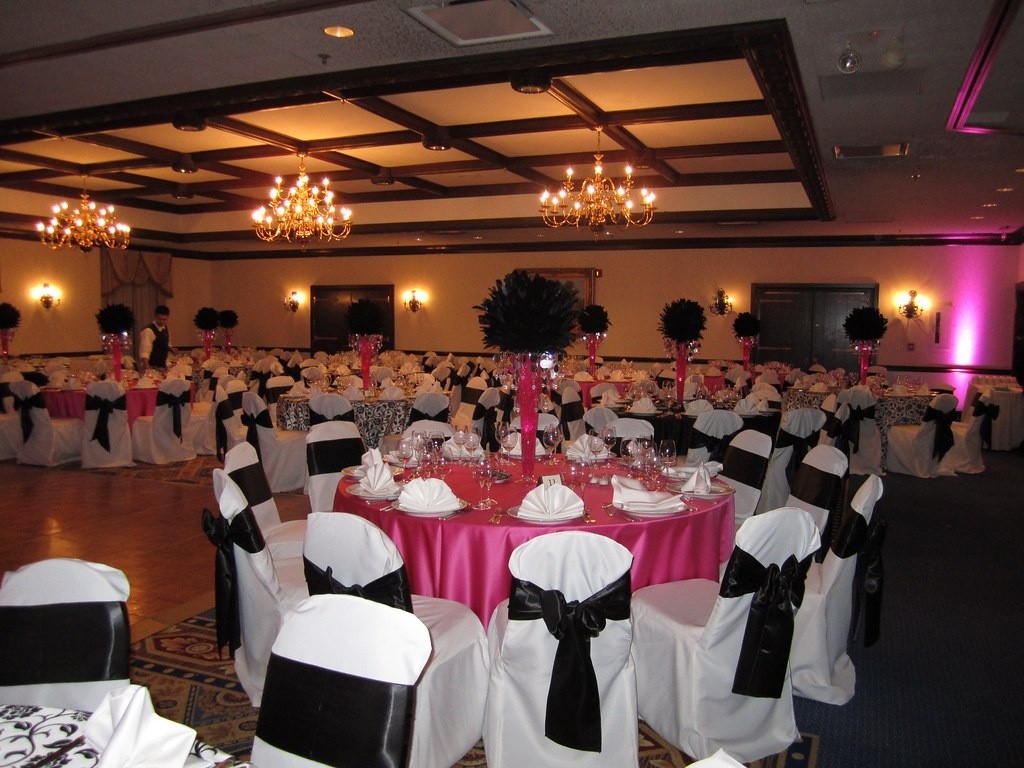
top-left (0, 454), bottom-right (224, 486)
top-left (131, 606), bottom-right (820, 768)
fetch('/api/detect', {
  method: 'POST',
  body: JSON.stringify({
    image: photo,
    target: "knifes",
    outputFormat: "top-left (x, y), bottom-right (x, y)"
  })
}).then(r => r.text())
top-left (33, 734), bottom-right (86, 768)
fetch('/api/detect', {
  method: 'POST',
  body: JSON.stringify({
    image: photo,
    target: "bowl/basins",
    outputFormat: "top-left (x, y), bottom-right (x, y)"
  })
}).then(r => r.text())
top-left (971, 375), bottom-right (1017, 383)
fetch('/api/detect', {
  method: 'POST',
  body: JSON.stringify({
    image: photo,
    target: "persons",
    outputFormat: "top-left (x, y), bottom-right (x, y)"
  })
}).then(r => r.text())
top-left (140, 305), bottom-right (180, 371)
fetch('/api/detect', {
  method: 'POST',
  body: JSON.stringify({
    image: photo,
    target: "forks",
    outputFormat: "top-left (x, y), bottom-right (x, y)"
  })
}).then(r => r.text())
top-left (602, 503), bottom-right (636, 523)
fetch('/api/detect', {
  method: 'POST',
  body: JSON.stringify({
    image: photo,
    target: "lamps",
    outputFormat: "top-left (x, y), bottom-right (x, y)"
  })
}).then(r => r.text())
top-left (284, 291), bottom-right (299, 312)
top-left (899, 290), bottom-right (923, 320)
top-left (403, 290), bottom-right (422, 312)
top-left (40, 284), bottom-right (60, 311)
top-left (836, 50), bottom-right (861, 75)
top-left (541, 124), bottom-right (658, 242)
top-left (709, 288), bottom-right (733, 315)
top-left (252, 149), bottom-right (353, 252)
top-left (37, 172), bottom-right (130, 256)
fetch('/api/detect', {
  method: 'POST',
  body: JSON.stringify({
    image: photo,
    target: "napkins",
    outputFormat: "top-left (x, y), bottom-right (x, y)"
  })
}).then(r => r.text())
top-left (681, 462), bottom-right (711, 494)
top-left (359, 463), bottom-right (399, 496)
top-left (355, 447), bottom-right (383, 476)
top-left (383, 451), bottom-right (416, 462)
top-left (661, 461), bottom-right (723, 479)
top-left (566, 434), bottom-right (608, 460)
top-left (611, 475), bottom-right (686, 513)
top-left (503, 432), bottom-right (545, 456)
top-left (398, 477), bottom-right (462, 510)
top-left (442, 436), bottom-right (484, 460)
top-left (517, 484), bottom-right (585, 519)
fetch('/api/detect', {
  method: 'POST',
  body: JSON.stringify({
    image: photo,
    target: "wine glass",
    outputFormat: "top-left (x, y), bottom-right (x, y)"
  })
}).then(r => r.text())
top-left (397, 423), bottom-right (676, 509)
top-left (0, 346), bottom-right (923, 414)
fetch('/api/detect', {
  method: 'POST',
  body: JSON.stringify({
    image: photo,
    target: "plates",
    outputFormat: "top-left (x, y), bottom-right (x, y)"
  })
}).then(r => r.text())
top-left (341, 449), bottom-right (736, 526)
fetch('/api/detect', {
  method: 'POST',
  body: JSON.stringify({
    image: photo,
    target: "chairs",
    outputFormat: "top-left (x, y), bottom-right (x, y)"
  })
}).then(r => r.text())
top-left (0, 351), bottom-right (1024, 766)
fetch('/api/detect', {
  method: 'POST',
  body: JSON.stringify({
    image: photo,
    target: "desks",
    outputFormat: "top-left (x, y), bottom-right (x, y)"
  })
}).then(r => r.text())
top-left (41, 381), bottom-right (194, 432)
top-left (782, 389), bottom-right (939, 471)
top-left (332, 456), bottom-right (736, 636)
top-left (548, 375), bottom-right (632, 407)
top-left (276, 392), bottom-right (452, 450)
top-left (585, 398), bottom-right (781, 456)
